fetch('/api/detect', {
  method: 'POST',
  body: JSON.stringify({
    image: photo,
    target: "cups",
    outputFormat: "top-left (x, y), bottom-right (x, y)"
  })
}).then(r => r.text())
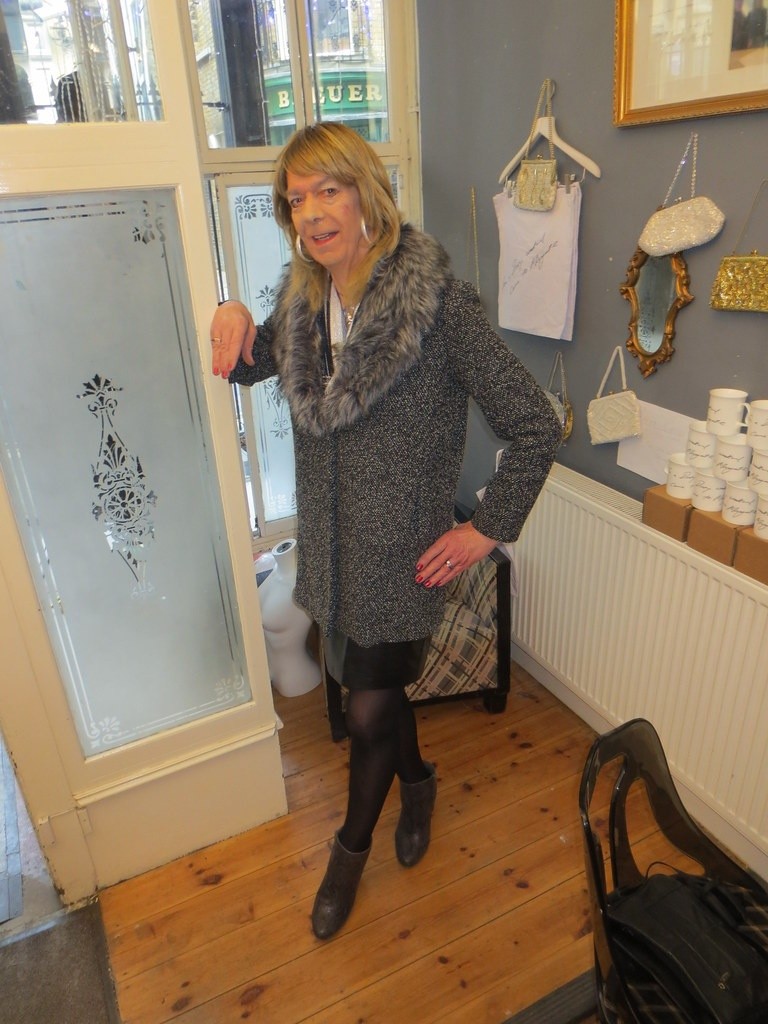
top-left (665, 390), bottom-right (768, 538)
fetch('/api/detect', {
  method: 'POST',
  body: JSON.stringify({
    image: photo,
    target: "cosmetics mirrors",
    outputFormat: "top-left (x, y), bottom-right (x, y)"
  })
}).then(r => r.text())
top-left (617, 206), bottom-right (696, 382)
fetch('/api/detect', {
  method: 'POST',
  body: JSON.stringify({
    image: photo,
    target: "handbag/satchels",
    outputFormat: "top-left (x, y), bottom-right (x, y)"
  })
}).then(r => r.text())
top-left (638, 133), bottom-right (726, 258)
top-left (538, 352), bottom-right (573, 440)
top-left (587, 347), bottom-right (640, 445)
top-left (514, 79), bottom-right (557, 212)
top-left (709, 179), bottom-right (768, 312)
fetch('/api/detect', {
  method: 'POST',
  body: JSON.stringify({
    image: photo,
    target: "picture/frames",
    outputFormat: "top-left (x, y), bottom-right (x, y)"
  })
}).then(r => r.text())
top-left (610, 0), bottom-right (768, 132)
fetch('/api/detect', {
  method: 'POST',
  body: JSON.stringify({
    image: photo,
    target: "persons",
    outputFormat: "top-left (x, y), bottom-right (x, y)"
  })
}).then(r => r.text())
top-left (209, 120), bottom-right (565, 940)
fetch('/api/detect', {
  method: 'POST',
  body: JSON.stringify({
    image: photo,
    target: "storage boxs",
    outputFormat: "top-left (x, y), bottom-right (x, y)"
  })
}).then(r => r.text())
top-left (640, 481), bottom-right (768, 587)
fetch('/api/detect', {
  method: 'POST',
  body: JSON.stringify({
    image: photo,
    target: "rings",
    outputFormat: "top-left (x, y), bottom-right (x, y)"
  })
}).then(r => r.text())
top-left (445, 560), bottom-right (454, 570)
top-left (211, 337), bottom-right (221, 343)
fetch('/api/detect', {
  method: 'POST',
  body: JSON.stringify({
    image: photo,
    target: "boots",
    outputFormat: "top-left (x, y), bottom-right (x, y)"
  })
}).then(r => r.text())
top-left (311, 830), bottom-right (368, 938)
top-left (394, 760), bottom-right (437, 866)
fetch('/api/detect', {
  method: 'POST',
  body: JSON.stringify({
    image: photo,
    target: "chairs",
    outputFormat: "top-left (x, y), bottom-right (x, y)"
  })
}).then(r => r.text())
top-left (314, 498), bottom-right (512, 742)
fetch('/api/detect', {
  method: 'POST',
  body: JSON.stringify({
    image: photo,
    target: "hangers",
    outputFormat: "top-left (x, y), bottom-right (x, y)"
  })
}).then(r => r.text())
top-left (496, 78), bottom-right (602, 194)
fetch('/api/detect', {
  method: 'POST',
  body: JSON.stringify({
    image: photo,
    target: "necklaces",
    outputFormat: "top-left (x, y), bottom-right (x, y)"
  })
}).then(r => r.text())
top-left (341, 306), bottom-right (354, 328)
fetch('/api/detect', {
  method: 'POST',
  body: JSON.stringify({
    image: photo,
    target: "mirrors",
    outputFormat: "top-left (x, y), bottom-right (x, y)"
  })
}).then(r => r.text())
top-left (616, 204), bottom-right (697, 379)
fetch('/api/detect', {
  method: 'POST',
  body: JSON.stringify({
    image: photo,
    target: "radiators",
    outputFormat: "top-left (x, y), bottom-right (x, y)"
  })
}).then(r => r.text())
top-left (496, 448), bottom-right (768, 890)
